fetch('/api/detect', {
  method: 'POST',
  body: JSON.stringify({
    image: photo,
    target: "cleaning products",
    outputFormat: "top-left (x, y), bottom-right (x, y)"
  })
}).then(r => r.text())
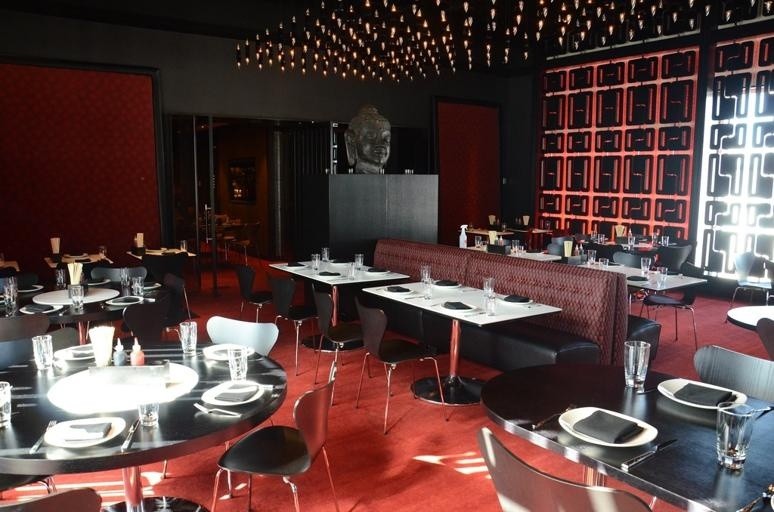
top-left (459, 224), bottom-right (469, 248)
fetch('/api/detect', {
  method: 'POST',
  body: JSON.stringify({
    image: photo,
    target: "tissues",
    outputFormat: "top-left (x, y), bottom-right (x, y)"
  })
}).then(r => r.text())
top-left (521, 216), bottom-right (530, 229)
top-left (487, 231), bottom-right (511, 255)
top-left (131, 232), bottom-right (145, 256)
top-left (66, 262), bottom-right (88, 298)
top-left (615, 224), bottom-right (628, 244)
top-left (561, 241), bottom-right (581, 265)
top-left (87, 325), bottom-right (116, 374)
top-left (488, 215), bottom-right (501, 231)
top-left (50, 237), bottom-right (62, 263)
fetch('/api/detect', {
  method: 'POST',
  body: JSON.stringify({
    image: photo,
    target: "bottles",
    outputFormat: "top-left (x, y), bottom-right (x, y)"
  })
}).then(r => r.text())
top-left (574, 243), bottom-right (584, 256)
top-left (114, 337), bottom-right (146, 365)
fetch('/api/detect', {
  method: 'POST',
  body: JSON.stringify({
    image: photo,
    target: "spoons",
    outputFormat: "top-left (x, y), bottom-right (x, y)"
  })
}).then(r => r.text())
top-left (738, 405), bottom-right (774, 429)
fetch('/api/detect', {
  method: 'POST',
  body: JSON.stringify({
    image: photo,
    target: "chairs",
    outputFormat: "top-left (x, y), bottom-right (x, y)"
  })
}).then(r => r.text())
top-left (459, 222), bottom-right (708, 350)
top-left (0, 245), bottom-right (334, 511)
top-left (478, 252), bottom-right (774, 512)
top-left (354, 292), bottom-right (448, 435)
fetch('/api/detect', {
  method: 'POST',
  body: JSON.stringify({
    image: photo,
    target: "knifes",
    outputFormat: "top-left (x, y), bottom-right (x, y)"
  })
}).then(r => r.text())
top-left (619, 436), bottom-right (682, 472)
top-left (121, 414), bottom-right (141, 453)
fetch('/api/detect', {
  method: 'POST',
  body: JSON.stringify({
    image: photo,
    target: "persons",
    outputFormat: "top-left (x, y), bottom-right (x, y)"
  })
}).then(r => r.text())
top-left (343, 102), bottom-right (393, 174)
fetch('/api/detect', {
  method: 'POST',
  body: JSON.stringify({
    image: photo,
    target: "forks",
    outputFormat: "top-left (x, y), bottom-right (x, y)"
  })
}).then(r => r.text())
top-left (733, 481), bottom-right (773, 511)
top-left (29, 420), bottom-right (55, 453)
top-left (193, 403), bottom-right (241, 417)
top-left (527, 401), bottom-right (578, 429)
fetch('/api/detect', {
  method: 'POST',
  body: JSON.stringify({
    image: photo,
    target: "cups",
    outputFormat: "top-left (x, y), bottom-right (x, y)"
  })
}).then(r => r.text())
top-left (311, 248), bottom-right (365, 279)
top-left (411, 266), bottom-right (503, 315)
top-left (227, 342), bottom-right (248, 380)
top-left (181, 238), bottom-right (190, 254)
top-left (53, 268), bottom-right (86, 316)
top-left (623, 340), bottom-right (651, 391)
top-left (583, 231), bottom-right (670, 287)
top-left (120, 267), bottom-right (146, 294)
top-left (137, 389), bottom-right (161, 427)
top-left (4, 275), bottom-right (20, 307)
top-left (712, 401), bottom-right (755, 475)
top-left (472, 214), bottom-right (551, 255)
top-left (97, 242), bottom-right (108, 260)
top-left (32, 334), bottom-right (53, 370)
top-left (88, 327), bottom-right (115, 370)
top-left (179, 322), bottom-right (199, 356)
top-left (0, 381), bottom-right (13, 429)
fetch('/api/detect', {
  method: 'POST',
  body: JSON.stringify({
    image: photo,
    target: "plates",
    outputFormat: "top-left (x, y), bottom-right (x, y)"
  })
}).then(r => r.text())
top-left (18, 284), bottom-right (44, 293)
top-left (201, 380), bottom-right (266, 406)
top-left (503, 293), bottom-right (533, 305)
top-left (658, 378), bottom-right (749, 411)
top-left (206, 342), bottom-right (256, 360)
top-left (363, 266), bottom-right (390, 275)
top-left (147, 246), bottom-right (181, 254)
top-left (106, 294), bottom-right (145, 305)
top-left (42, 414), bottom-right (126, 449)
top-left (19, 302), bottom-right (63, 314)
top-left (382, 287), bottom-right (414, 293)
top-left (65, 251), bottom-right (93, 264)
top-left (560, 405), bottom-right (660, 447)
top-left (88, 279), bottom-right (111, 286)
top-left (54, 344), bottom-right (111, 361)
top-left (0, 295), bottom-right (12, 305)
top-left (280, 263), bottom-right (309, 268)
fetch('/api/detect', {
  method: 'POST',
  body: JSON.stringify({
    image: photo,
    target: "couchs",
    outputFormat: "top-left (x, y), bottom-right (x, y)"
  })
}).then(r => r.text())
top-left (353, 237), bottom-right (662, 374)
